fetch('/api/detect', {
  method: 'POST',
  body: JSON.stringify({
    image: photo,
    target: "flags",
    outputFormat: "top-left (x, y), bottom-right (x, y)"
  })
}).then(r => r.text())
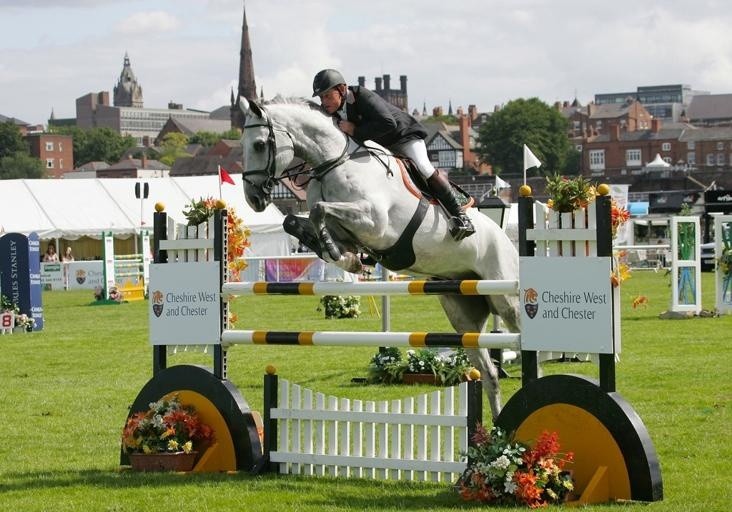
top-left (220, 166), bottom-right (235, 185)
top-left (495, 175), bottom-right (511, 189)
top-left (524, 144), bottom-right (541, 171)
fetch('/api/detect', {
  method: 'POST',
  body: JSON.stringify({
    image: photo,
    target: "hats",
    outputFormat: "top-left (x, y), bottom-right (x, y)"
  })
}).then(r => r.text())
top-left (312, 68), bottom-right (346, 98)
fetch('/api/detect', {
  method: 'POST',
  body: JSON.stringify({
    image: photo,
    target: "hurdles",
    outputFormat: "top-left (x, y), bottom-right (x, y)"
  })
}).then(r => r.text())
top-left (102, 228), bottom-right (153, 302)
top-left (120, 183), bottom-right (664, 503)
top-left (700, 214), bottom-right (732, 316)
top-left (621, 213), bottom-right (708, 319)
top-left (370, 255), bottom-right (523, 382)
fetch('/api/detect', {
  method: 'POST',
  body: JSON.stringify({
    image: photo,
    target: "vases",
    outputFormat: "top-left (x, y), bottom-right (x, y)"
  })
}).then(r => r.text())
top-left (403, 372), bottom-right (441, 386)
top-left (127, 450), bottom-right (199, 474)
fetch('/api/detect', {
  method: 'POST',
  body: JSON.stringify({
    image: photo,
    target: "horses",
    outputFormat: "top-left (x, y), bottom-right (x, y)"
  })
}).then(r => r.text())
top-left (237, 94), bottom-right (520, 423)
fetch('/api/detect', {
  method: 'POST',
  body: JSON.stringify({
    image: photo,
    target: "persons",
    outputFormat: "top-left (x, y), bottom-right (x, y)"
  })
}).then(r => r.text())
top-left (41, 244), bottom-right (58, 262)
top-left (308, 70), bottom-right (475, 268)
top-left (61, 246), bottom-right (76, 263)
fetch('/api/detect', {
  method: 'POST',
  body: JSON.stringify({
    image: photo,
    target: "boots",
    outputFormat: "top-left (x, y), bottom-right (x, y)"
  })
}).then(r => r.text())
top-left (426, 168), bottom-right (477, 241)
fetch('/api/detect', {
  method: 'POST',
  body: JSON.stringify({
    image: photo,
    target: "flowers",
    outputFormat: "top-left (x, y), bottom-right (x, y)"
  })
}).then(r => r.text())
top-left (545, 174), bottom-right (631, 285)
top-left (369, 347), bottom-right (480, 382)
top-left (189, 192), bottom-right (250, 285)
top-left (119, 396), bottom-right (215, 452)
top-left (456, 418), bottom-right (577, 508)
